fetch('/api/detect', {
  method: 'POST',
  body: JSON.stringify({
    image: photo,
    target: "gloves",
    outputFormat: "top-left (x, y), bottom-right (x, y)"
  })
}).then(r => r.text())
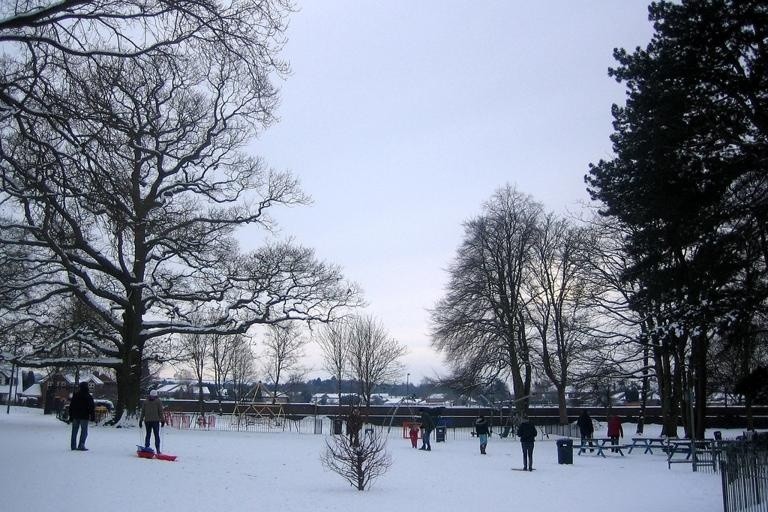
top-left (139, 420), bottom-right (142, 428)
top-left (161, 422), bottom-right (165, 428)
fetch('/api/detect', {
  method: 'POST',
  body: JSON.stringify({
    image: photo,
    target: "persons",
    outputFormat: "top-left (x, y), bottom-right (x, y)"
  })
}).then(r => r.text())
top-left (476, 415), bottom-right (490, 454)
top-left (138, 390), bottom-right (165, 454)
top-left (518, 416), bottom-right (537, 471)
top-left (67, 382), bottom-right (95, 451)
top-left (608, 415), bottom-right (623, 453)
top-left (577, 409), bottom-right (594, 453)
top-left (410, 408), bottom-right (435, 451)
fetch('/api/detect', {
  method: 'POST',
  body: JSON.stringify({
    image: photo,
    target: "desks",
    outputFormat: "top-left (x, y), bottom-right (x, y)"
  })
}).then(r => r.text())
top-left (627, 437), bottom-right (666, 454)
top-left (669, 438), bottom-right (715, 462)
top-left (578, 437), bottom-right (624, 458)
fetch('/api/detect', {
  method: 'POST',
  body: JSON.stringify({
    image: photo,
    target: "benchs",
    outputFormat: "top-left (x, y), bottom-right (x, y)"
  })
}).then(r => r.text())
top-left (625, 444), bottom-right (664, 449)
top-left (579, 445), bottom-right (623, 451)
top-left (666, 448), bottom-right (716, 456)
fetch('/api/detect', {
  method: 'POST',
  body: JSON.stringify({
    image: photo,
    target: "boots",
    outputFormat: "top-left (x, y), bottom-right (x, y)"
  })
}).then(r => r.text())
top-left (419, 445), bottom-right (431, 451)
top-left (523, 464), bottom-right (532, 472)
top-left (481, 448), bottom-right (486, 454)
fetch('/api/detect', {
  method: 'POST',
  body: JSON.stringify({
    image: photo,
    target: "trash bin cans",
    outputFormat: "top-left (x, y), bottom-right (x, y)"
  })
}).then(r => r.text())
top-left (334, 419), bottom-right (342, 434)
top-left (95, 406), bottom-right (108, 426)
top-left (714, 431), bottom-right (721, 440)
top-left (557, 438), bottom-right (573, 464)
top-left (436, 426), bottom-right (445, 442)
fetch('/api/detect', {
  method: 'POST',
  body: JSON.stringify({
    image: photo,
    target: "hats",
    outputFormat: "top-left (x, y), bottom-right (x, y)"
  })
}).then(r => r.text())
top-left (150, 390), bottom-right (157, 396)
